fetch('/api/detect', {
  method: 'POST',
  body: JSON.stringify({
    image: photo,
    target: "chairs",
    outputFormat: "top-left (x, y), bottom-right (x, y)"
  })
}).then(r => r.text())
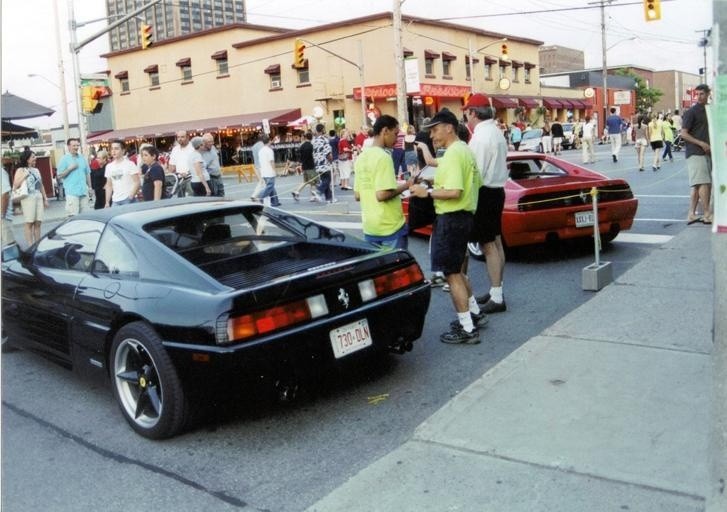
top-left (506, 160), bottom-right (530, 181)
top-left (186, 222), bottom-right (232, 266)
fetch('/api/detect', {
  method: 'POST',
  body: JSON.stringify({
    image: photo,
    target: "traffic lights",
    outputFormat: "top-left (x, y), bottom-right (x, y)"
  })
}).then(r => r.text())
top-left (80, 85), bottom-right (102, 114)
top-left (141, 24), bottom-right (152, 49)
top-left (294, 38), bottom-right (305, 67)
top-left (643, 0), bottom-right (660, 22)
top-left (500, 43), bottom-right (508, 60)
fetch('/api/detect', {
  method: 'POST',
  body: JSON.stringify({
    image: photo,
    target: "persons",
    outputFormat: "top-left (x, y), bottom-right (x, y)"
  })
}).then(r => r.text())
top-left (681, 84), bottom-right (713, 225)
top-left (495, 112), bottom-right (598, 164)
top-left (255, 133), bottom-right (282, 207)
top-left (279, 107), bottom-right (474, 204)
top-left (91, 131), bottom-right (223, 209)
top-left (56, 138), bottom-right (92, 216)
top-left (407, 111), bottom-right (489, 344)
top-left (250, 133), bottom-right (265, 202)
top-left (13, 150), bottom-right (50, 247)
top-left (458, 94), bottom-right (507, 314)
top-left (601, 108), bottom-right (683, 172)
top-left (353, 115), bottom-right (415, 252)
top-left (1, 169), bottom-right (16, 247)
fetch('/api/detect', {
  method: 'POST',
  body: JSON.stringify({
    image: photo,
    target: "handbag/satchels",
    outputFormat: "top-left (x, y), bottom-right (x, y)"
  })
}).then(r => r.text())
top-left (11, 166), bottom-right (28, 201)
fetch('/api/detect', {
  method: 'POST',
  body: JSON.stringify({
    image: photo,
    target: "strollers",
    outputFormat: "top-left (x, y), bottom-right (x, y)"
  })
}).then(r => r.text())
top-left (159, 173), bottom-right (190, 198)
top-left (671, 134), bottom-right (685, 151)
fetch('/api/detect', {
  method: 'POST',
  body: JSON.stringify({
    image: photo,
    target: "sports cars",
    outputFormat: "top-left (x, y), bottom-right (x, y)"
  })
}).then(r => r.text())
top-left (397, 150), bottom-right (639, 260)
top-left (1, 197), bottom-right (435, 440)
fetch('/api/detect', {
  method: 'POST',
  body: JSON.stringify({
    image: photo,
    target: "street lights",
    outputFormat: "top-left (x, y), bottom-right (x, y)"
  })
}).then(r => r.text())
top-left (602, 36), bottom-right (638, 131)
top-left (26, 73), bottom-right (73, 105)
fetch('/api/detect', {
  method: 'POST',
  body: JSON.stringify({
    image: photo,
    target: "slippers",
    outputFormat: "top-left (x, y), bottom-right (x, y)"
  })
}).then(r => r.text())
top-left (688, 216), bottom-right (712, 225)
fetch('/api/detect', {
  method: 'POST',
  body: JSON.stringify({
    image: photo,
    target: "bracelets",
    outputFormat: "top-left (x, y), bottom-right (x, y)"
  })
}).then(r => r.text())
top-left (44, 198), bottom-right (49, 200)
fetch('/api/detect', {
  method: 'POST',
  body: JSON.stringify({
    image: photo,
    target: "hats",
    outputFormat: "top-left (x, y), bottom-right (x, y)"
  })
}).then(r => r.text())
top-left (190, 136), bottom-right (204, 149)
top-left (460, 93), bottom-right (490, 111)
top-left (422, 111), bottom-right (459, 129)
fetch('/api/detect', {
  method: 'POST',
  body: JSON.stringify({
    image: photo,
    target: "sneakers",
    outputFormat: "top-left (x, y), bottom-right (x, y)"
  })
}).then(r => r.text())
top-left (612, 154), bottom-right (618, 162)
top-left (479, 300), bottom-right (506, 314)
top-left (327, 198), bottom-right (338, 204)
top-left (476, 293), bottom-right (505, 304)
top-left (450, 312), bottom-right (488, 328)
top-left (428, 275), bottom-right (469, 291)
top-left (310, 190), bottom-right (324, 203)
top-left (292, 191), bottom-right (301, 202)
top-left (440, 325), bottom-right (481, 345)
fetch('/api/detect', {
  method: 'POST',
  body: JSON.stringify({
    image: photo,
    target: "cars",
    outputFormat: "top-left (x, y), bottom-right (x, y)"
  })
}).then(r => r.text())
top-left (510, 121), bottom-right (588, 153)
